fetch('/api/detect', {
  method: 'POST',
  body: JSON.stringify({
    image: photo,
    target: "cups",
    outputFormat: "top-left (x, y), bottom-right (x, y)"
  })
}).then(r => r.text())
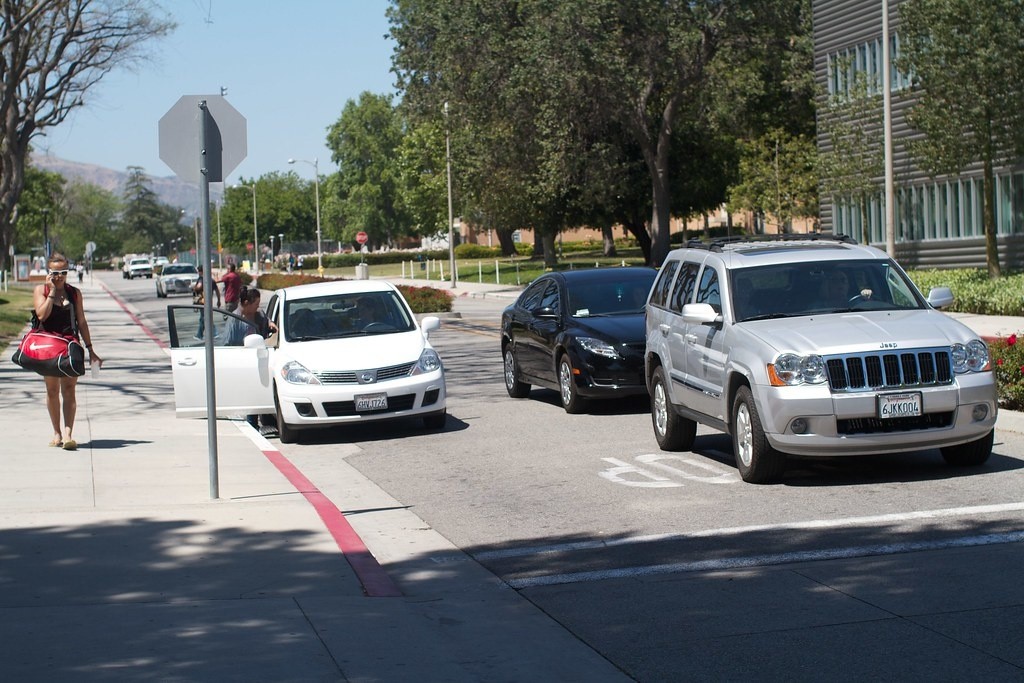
top-left (91, 360), bottom-right (100, 379)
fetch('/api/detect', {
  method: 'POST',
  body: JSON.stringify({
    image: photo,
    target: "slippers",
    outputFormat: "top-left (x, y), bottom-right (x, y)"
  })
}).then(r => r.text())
top-left (62, 438), bottom-right (77, 449)
top-left (48, 439), bottom-right (63, 447)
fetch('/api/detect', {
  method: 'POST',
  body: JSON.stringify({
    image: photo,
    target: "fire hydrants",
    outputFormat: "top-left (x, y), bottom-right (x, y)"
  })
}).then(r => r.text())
top-left (318, 266), bottom-right (325, 279)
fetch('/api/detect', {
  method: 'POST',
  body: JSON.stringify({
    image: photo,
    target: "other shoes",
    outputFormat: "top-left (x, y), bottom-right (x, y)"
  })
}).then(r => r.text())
top-left (192, 334), bottom-right (202, 340)
top-left (213, 334), bottom-right (219, 338)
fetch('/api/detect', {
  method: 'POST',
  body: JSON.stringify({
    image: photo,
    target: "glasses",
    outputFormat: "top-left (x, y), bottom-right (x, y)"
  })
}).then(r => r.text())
top-left (47, 269), bottom-right (68, 276)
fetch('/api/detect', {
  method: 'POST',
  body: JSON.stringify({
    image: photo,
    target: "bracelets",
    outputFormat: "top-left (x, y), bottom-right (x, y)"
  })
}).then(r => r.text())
top-left (85, 344), bottom-right (92, 348)
top-left (49, 294), bottom-right (55, 299)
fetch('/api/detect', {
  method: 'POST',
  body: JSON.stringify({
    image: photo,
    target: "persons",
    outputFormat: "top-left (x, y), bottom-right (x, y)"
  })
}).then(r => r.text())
top-left (216, 263), bottom-right (240, 320)
top-left (33, 254), bottom-right (103, 451)
top-left (223, 288), bottom-right (278, 433)
top-left (192, 265), bottom-right (221, 341)
top-left (76, 261), bottom-right (84, 283)
top-left (840, 270), bottom-right (872, 300)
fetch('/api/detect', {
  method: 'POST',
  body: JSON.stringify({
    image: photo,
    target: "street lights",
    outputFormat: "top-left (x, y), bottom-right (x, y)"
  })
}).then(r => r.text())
top-left (442, 100), bottom-right (461, 290)
top-left (232, 184), bottom-right (259, 275)
top-left (287, 158), bottom-right (322, 267)
top-left (269, 236), bottom-right (275, 273)
top-left (279, 234), bottom-right (284, 255)
top-left (41, 207), bottom-right (50, 250)
top-left (181, 209), bottom-right (199, 268)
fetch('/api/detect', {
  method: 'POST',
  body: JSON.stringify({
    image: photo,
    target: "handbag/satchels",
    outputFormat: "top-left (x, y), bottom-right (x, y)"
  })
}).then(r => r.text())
top-left (11, 327), bottom-right (85, 377)
top-left (193, 295), bottom-right (204, 305)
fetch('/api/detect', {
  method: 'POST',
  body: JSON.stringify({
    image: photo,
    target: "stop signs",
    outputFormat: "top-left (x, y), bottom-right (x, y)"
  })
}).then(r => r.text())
top-left (357, 232), bottom-right (368, 243)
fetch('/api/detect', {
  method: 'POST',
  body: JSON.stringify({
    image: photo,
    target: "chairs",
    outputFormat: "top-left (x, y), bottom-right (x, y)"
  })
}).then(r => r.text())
top-left (292, 307), bottom-right (325, 335)
top-left (349, 297), bottom-right (388, 326)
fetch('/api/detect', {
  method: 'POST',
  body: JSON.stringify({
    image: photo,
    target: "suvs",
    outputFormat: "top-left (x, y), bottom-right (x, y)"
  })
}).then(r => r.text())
top-left (642, 234), bottom-right (1000, 486)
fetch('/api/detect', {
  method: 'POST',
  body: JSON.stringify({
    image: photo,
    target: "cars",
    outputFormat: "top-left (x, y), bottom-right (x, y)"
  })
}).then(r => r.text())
top-left (122, 257), bottom-right (152, 280)
top-left (150, 256), bottom-right (168, 265)
top-left (155, 261), bottom-right (199, 299)
top-left (166, 280), bottom-right (448, 445)
top-left (500, 265), bottom-right (659, 415)
